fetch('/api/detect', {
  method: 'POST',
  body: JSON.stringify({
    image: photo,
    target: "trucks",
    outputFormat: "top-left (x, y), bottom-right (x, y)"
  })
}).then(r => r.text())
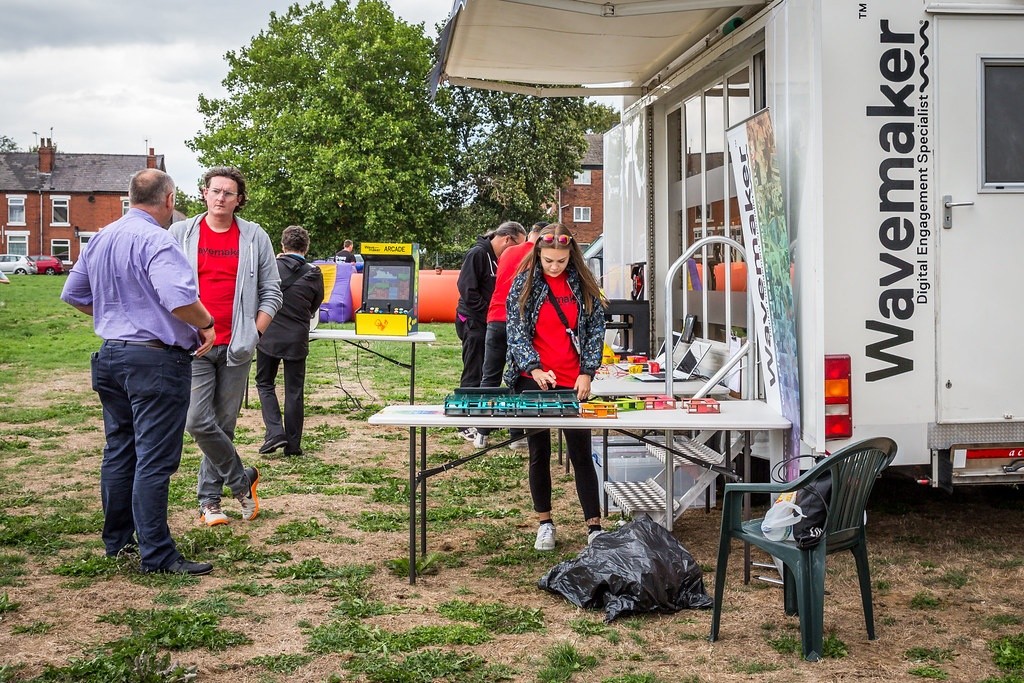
top-left (431, 1), bottom-right (1024, 509)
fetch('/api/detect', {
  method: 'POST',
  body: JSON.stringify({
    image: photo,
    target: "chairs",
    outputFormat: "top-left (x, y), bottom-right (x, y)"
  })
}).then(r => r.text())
top-left (710, 437), bottom-right (899, 661)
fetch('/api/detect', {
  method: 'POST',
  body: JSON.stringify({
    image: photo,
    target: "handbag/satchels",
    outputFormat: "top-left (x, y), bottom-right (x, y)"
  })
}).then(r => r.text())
top-left (567, 328), bottom-right (581, 355)
top-left (769, 455), bottom-right (866, 550)
top-left (761, 491), bottom-right (804, 541)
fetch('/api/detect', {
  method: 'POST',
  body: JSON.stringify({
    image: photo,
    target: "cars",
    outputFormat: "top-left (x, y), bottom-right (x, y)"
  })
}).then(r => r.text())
top-left (0, 254), bottom-right (38, 276)
top-left (28, 255), bottom-right (65, 276)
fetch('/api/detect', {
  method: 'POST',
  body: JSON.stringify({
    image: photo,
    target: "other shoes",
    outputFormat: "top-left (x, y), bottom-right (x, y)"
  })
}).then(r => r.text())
top-left (474, 432), bottom-right (487, 448)
top-left (457, 427), bottom-right (478, 441)
top-left (509, 438), bottom-right (529, 449)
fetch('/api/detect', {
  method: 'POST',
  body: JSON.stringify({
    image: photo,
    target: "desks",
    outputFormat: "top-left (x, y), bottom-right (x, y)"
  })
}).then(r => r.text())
top-left (246, 329), bottom-right (436, 405)
top-left (591, 364), bottom-right (731, 518)
top-left (368, 400), bottom-right (792, 585)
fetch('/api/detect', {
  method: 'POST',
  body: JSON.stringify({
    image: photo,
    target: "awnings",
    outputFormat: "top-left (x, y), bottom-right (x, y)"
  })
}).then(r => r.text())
top-left (425, 0), bottom-right (773, 105)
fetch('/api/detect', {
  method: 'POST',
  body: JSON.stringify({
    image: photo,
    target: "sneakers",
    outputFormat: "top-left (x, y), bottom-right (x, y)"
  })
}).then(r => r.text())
top-left (284, 447), bottom-right (302, 456)
top-left (534, 522), bottom-right (556, 549)
top-left (237, 466), bottom-right (259, 520)
top-left (199, 501), bottom-right (228, 526)
top-left (259, 434), bottom-right (288, 454)
top-left (587, 530), bottom-right (605, 544)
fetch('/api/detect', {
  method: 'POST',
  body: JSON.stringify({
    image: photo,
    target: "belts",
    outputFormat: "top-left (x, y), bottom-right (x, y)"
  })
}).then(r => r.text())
top-left (106, 338), bottom-right (183, 350)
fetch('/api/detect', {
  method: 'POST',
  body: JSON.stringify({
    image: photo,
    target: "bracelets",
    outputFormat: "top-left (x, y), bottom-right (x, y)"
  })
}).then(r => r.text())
top-left (258, 330), bottom-right (262, 338)
top-left (204, 317), bottom-right (214, 329)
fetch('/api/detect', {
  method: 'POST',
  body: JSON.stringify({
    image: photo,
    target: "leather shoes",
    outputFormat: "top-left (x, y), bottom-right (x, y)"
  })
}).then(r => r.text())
top-left (143, 555), bottom-right (213, 575)
top-left (105, 537), bottom-right (141, 559)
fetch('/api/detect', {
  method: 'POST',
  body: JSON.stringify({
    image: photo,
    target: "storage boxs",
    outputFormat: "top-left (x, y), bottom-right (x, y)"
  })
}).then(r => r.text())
top-left (592, 435), bottom-right (718, 511)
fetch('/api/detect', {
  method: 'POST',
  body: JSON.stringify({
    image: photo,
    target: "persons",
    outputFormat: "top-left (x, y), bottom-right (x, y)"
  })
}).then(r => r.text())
top-left (455, 221), bottom-right (526, 440)
top-left (255, 226), bottom-right (324, 454)
top-left (169, 166), bottom-right (282, 526)
top-left (475, 222), bottom-right (548, 448)
top-left (334, 240), bottom-right (357, 263)
top-left (61, 168), bottom-right (215, 577)
top-left (505, 224), bottom-right (606, 549)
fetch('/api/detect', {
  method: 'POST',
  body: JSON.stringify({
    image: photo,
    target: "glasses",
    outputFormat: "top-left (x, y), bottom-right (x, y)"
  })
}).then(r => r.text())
top-left (539, 233), bottom-right (573, 246)
top-left (206, 188), bottom-right (239, 200)
